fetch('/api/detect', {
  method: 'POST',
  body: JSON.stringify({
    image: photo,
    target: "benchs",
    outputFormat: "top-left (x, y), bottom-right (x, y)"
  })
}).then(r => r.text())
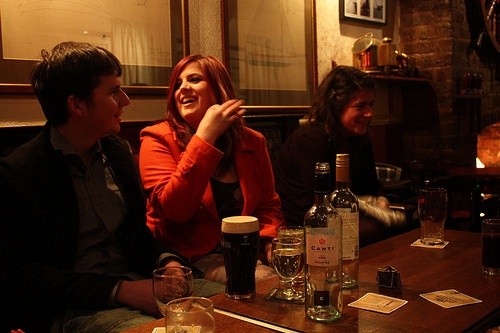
top-left (0, 112), bottom-right (303, 193)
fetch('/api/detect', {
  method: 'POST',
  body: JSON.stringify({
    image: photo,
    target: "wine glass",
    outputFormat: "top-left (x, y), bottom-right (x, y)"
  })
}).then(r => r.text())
top-left (277, 226), bottom-right (305, 291)
top-left (271, 237), bottom-right (306, 303)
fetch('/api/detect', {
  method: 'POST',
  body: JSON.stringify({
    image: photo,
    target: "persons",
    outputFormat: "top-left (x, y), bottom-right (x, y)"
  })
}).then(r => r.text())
top-left (278, 67), bottom-right (414, 249)
top-left (138, 56), bottom-right (286, 286)
top-left (0, 42), bottom-right (226, 333)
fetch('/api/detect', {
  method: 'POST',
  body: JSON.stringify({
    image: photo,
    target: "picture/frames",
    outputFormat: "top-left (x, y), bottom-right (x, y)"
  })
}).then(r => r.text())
top-left (339, 0), bottom-right (389, 26)
top-left (0, 0), bottom-right (191, 96)
top-left (220, 0), bottom-right (319, 116)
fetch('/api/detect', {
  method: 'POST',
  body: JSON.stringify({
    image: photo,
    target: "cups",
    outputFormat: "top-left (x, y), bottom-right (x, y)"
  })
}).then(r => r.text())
top-left (418, 188), bottom-right (447, 246)
top-left (482, 218), bottom-right (500, 277)
top-left (153, 266), bottom-right (194, 333)
top-left (166, 297), bottom-right (216, 333)
top-left (376, 165), bottom-right (401, 184)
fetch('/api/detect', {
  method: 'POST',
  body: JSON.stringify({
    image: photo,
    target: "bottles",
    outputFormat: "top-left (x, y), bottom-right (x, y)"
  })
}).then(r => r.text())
top-left (329, 154), bottom-right (360, 290)
top-left (221, 216), bottom-right (260, 300)
top-left (304, 162), bottom-right (342, 323)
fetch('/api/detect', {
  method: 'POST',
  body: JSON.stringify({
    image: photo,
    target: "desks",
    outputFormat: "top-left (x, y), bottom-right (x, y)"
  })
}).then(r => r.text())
top-left (197, 225), bottom-right (500, 333)
top-left (110, 309), bottom-right (285, 333)
top-left (453, 93), bottom-right (498, 98)
